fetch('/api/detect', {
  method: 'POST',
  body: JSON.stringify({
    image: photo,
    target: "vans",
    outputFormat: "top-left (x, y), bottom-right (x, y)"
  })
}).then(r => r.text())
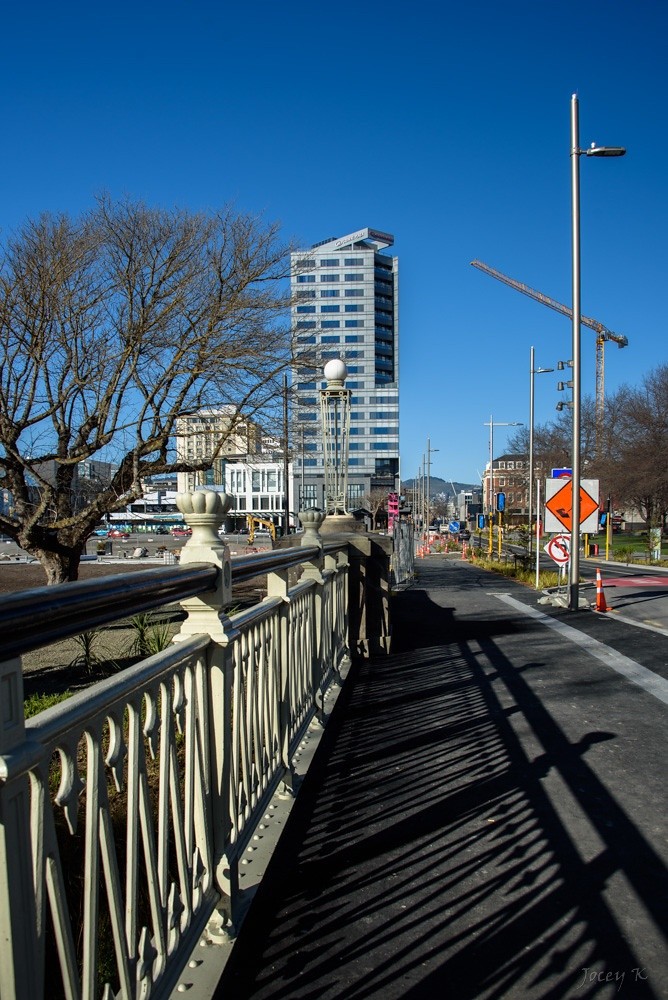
top-left (439, 524), bottom-right (450, 534)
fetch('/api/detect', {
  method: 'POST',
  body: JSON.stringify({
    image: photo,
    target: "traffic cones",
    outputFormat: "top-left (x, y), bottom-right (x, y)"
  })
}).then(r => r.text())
top-left (593, 567), bottom-right (613, 612)
top-left (416, 536), bottom-right (475, 561)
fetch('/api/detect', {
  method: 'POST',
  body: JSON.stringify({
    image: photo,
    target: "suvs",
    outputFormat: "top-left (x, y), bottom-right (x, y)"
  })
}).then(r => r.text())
top-left (156, 526), bottom-right (168, 535)
top-left (254, 529), bottom-right (270, 538)
top-left (420, 526), bottom-right (438, 538)
top-left (454, 530), bottom-right (471, 541)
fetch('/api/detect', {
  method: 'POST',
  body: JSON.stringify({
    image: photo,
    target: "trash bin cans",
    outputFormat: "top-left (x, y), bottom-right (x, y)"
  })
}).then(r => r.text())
top-left (594, 544), bottom-right (599, 556)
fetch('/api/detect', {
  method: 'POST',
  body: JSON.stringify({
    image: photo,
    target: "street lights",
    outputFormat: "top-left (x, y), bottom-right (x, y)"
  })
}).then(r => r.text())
top-left (528, 369), bottom-right (556, 555)
top-left (571, 145), bottom-right (628, 611)
top-left (490, 423), bottom-right (525, 512)
top-left (413, 450), bottom-right (438, 543)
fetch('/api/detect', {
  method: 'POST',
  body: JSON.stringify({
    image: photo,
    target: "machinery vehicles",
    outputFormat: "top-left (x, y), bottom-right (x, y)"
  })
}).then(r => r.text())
top-left (246, 512), bottom-right (296, 546)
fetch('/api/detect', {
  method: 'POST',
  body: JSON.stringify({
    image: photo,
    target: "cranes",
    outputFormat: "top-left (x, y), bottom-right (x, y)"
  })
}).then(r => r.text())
top-left (471, 260), bottom-right (629, 459)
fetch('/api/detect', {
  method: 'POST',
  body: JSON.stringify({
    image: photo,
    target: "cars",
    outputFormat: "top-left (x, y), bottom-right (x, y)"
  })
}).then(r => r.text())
top-left (239, 529), bottom-right (249, 535)
top-left (170, 528), bottom-right (192, 537)
top-left (218, 527), bottom-right (227, 535)
top-left (107, 530), bottom-right (130, 538)
top-left (93, 528), bottom-right (110, 536)
